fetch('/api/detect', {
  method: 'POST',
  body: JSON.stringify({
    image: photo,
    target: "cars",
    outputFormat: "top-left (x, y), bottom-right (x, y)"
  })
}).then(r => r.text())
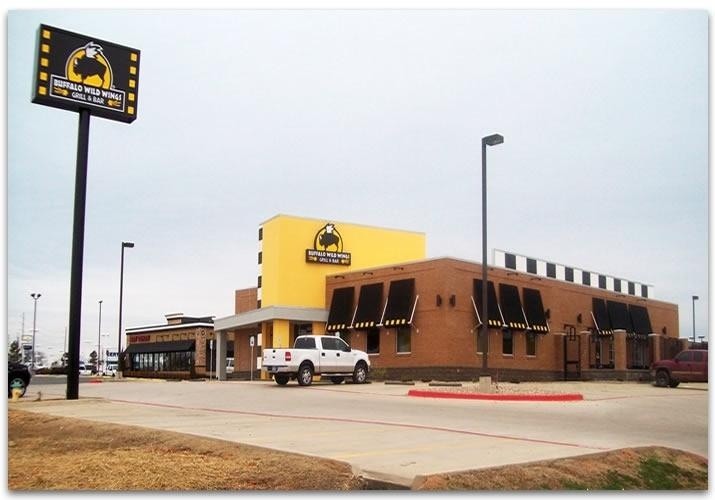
top-left (79, 363), bottom-right (118, 377)
top-left (8, 360), bottom-right (32, 400)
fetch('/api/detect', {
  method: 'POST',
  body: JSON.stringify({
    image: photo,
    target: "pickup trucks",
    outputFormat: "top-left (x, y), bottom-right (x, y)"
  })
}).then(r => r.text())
top-left (262, 334), bottom-right (371, 387)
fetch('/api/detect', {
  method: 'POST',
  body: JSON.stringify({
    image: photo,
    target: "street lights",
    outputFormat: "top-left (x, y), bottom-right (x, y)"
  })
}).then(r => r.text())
top-left (479, 133), bottom-right (505, 375)
top-left (692, 296), bottom-right (699, 341)
top-left (117, 242), bottom-right (136, 353)
top-left (98, 300), bottom-right (103, 369)
top-left (31, 294), bottom-right (41, 365)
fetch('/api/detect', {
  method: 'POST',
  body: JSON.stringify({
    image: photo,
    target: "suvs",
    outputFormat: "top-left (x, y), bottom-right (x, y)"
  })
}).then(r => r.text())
top-left (649, 349), bottom-right (707, 389)
